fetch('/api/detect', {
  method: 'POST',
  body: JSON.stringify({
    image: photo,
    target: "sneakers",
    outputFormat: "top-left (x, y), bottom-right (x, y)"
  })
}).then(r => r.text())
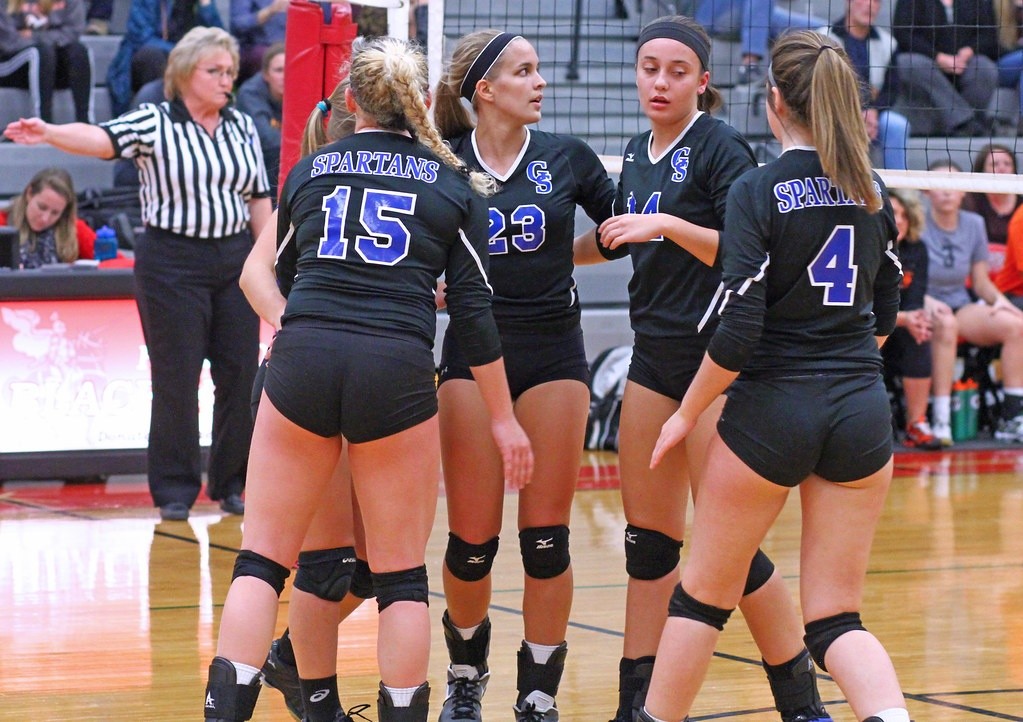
top-left (513, 691), bottom-right (568, 722)
top-left (437, 665), bottom-right (490, 722)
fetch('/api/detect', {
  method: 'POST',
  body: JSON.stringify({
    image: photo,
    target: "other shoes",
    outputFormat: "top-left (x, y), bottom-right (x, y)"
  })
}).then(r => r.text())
top-left (956, 120), bottom-right (981, 136)
top-left (219, 494), bottom-right (243, 514)
top-left (995, 417), bottom-right (1023, 442)
top-left (906, 418), bottom-right (937, 448)
top-left (607, 663), bottom-right (652, 722)
top-left (376, 682), bottom-right (430, 722)
top-left (160, 503), bottom-right (188, 520)
top-left (85, 18), bottom-right (108, 35)
top-left (769, 654), bottom-right (835, 722)
top-left (203, 657), bottom-right (262, 722)
top-left (299, 707), bottom-right (353, 722)
top-left (262, 640), bottom-right (305, 720)
top-left (933, 422), bottom-right (953, 445)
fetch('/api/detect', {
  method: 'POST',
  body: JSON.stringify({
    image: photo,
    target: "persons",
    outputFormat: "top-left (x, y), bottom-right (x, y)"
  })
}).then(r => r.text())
top-left (572, 15), bottom-right (834, 722)
top-left (0, 167), bottom-right (98, 271)
top-left (633, 28), bottom-right (914, 722)
top-left (5, 26), bottom-right (272, 521)
top-left (0, 0), bottom-right (332, 195)
top-left (675, 0), bottom-right (1023, 449)
top-left (205, 37), bottom-right (533, 722)
top-left (434, 28), bottom-right (617, 722)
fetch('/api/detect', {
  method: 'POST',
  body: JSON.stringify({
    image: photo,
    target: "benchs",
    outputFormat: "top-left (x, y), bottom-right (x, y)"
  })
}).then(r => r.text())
top-left (0, 0), bottom-right (1023, 305)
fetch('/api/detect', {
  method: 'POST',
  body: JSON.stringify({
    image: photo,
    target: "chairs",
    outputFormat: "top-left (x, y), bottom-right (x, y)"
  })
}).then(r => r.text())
top-left (956, 242), bottom-right (1008, 439)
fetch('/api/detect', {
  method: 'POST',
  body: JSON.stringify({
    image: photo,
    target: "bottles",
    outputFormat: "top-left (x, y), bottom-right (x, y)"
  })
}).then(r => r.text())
top-left (966, 378), bottom-right (981, 440)
top-left (93, 224), bottom-right (117, 262)
top-left (950, 380), bottom-right (967, 442)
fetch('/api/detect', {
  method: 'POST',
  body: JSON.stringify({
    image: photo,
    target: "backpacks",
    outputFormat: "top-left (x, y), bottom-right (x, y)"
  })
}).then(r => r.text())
top-left (584, 343), bottom-right (634, 451)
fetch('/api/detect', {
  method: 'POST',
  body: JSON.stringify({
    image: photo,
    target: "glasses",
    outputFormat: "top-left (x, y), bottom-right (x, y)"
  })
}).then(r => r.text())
top-left (206, 68), bottom-right (233, 75)
